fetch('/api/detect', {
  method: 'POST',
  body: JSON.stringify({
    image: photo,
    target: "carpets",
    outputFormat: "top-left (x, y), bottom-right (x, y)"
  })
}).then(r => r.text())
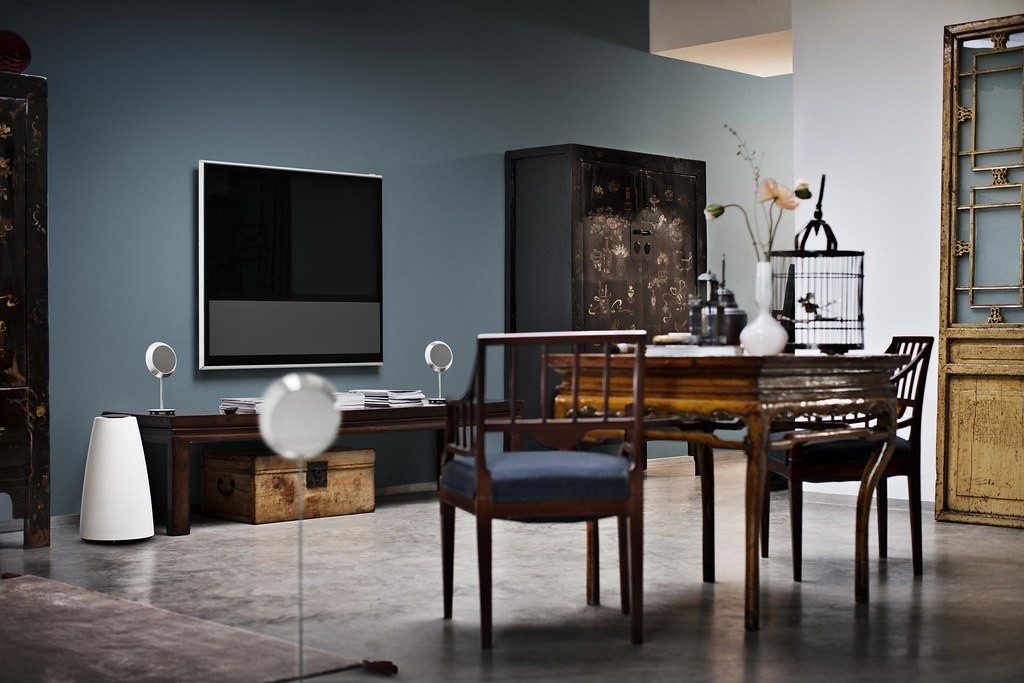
top-left (0, 573), bottom-right (367, 683)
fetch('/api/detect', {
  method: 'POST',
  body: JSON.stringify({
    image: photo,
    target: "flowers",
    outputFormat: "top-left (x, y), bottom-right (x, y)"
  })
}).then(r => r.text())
top-left (704, 122), bottom-right (815, 261)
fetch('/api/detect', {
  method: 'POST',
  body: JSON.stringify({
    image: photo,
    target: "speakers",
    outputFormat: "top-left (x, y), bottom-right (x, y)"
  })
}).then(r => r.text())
top-left (424, 340), bottom-right (454, 404)
top-left (145, 341), bottom-right (178, 415)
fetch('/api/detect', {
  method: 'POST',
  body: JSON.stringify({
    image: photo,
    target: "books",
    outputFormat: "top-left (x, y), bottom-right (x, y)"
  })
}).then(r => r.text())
top-left (220, 388), bottom-right (426, 414)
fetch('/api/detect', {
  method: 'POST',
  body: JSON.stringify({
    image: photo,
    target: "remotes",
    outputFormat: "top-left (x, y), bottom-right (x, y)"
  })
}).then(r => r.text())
top-left (224, 406), bottom-right (238, 414)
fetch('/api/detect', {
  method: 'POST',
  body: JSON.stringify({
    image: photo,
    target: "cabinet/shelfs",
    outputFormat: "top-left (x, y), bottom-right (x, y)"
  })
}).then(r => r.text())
top-left (504, 143), bottom-right (707, 456)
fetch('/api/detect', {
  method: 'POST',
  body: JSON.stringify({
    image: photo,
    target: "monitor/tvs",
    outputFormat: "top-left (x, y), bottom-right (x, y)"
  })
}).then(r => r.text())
top-left (198, 160), bottom-right (385, 370)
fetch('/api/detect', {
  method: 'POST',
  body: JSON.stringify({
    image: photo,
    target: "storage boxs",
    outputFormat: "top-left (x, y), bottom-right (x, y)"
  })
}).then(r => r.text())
top-left (200, 447), bottom-right (378, 525)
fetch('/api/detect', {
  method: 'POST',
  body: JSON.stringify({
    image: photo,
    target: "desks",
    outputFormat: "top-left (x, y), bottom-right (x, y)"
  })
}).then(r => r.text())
top-left (547, 349), bottom-right (913, 647)
top-left (100, 397), bottom-right (529, 538)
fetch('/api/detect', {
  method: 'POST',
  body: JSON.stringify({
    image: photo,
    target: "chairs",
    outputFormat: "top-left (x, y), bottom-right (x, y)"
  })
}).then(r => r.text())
top-left (760, 335), bottom-right (936, 603)
top-left (436, 329), bottom-right (648, 652)
top-left (0, 68), bottom-right (51, 550)
top-left (0, 386), bottom-right (34, 550)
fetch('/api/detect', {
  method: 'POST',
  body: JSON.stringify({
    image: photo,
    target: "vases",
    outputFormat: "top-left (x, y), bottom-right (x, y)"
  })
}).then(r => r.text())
top-left (739, 260), bottom-right (790, 356)
